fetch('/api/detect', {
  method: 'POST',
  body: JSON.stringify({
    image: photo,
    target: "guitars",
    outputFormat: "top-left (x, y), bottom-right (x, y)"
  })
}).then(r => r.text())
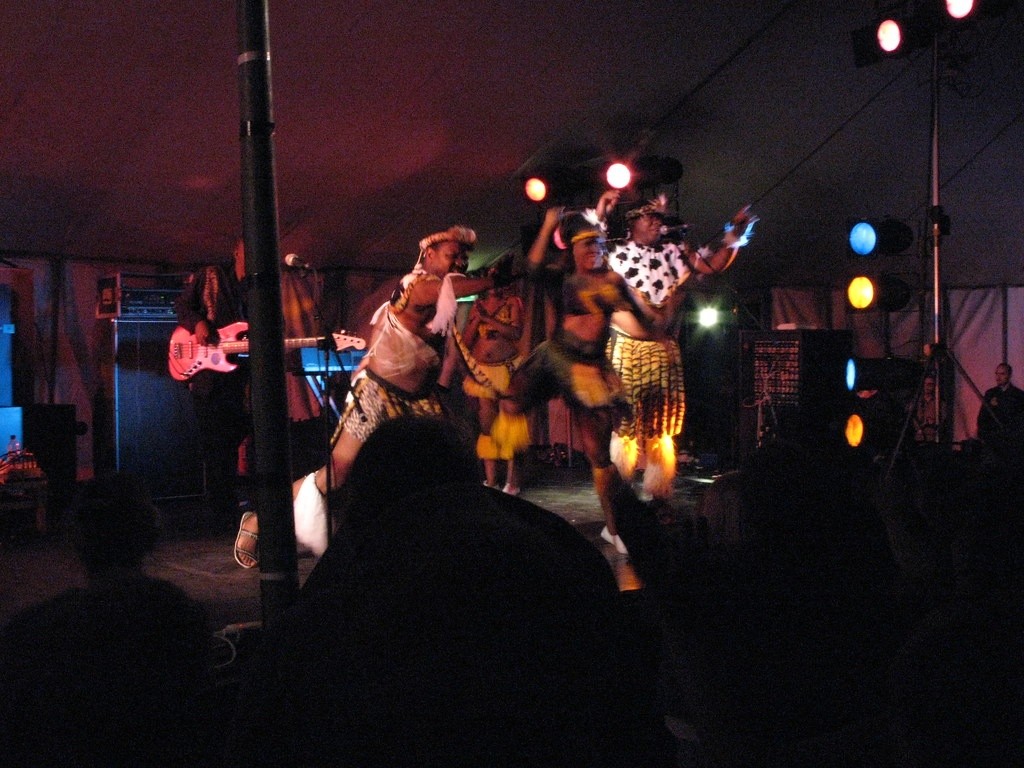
top-left (168, 320), bottom-right (367, 382)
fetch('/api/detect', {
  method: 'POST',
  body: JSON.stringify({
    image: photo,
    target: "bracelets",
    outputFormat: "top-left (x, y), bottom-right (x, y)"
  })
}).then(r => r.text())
top-left (582, 205), bottom-right (608, 231)
top-left (723, 231), bottom-right (750, 250)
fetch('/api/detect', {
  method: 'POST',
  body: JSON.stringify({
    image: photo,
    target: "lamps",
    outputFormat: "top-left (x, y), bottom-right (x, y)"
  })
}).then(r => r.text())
top-left (524, 167), bottom-right (587, 205)
top-left (843, 271), bottom-right (929, 317)
top-left (633, 153), bottom-right (683, 191)
top-left (844, 355), bottom-right (926, 396)
top-left (845, 215), bottom-right (929, 258)
top-left (836, 411), bottom-right (928, 454)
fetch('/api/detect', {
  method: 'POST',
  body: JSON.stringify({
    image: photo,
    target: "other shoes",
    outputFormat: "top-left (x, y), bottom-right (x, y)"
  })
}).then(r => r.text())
top-left (482, 480), bottom-right (502, 491)
top-left (504, 484), bottom-right (522, 496)
top-left (601, 526), bottom-right (630, 555)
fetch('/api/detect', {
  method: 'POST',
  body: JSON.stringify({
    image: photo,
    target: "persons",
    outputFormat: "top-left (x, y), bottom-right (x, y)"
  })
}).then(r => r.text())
top-left (1, 144), bottom-right (1021, 768)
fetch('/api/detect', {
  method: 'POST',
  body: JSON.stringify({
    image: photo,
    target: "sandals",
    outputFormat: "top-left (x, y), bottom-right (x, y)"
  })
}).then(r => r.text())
top-left (233, 512), bottom-right (262, 570)
